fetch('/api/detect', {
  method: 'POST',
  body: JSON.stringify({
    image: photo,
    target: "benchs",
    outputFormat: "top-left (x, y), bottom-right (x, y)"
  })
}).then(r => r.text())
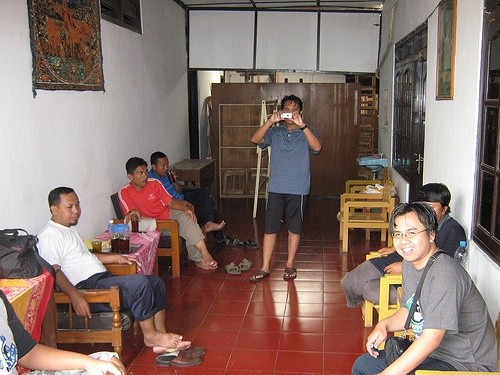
top-left (111, 189), bottom-right (208, 275)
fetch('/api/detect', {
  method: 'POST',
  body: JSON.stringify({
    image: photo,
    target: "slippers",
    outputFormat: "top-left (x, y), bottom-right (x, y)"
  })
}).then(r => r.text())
top-left (155, 349), bottom-right (202, 367)
top-left (163, 347), bottom-right (206, 356)
top-left (249, 270), bottom-right (270, 281)
top-left (283, 268), bottom-right (297, 280)
top-left (239, 259), bottom-right (254, 270)
top-left (225, 262), bottom-right (242, 275)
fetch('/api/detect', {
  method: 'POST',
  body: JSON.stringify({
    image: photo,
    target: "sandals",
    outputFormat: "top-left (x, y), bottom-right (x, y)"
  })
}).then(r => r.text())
top-left (238, 240), bottom-right (258, 249)
top-left (217, 235), bottom-right (240, 247)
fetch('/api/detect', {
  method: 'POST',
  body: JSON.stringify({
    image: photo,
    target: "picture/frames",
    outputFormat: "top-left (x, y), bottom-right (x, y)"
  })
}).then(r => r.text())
top-left (435, 0), bottom-right (458, 101)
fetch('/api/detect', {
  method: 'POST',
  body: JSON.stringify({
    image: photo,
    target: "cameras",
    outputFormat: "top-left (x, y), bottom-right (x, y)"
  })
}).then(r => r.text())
top-left (280, 113), bottom-right (292, 119)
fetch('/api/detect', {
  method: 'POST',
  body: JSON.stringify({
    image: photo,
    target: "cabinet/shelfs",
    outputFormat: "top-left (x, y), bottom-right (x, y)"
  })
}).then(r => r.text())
top-left (210, 82), bottom-right (375, 202)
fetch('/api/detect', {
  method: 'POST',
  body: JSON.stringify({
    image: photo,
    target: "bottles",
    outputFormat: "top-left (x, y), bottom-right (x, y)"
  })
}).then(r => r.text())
top-left (454, 241), bottom-right (468, 268)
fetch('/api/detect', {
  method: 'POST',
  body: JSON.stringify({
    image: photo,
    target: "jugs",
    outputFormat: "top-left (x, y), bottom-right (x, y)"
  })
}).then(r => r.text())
top-left (111, 225), bottom-right (129, 238)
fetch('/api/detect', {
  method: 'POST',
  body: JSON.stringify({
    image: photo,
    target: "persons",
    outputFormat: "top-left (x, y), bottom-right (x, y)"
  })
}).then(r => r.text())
top-left (32, 186), bottom-right (192, 354)
top-left (341, 183), bottom-right (466, 321)
top-left (352, 202), bottom-right (497, 375)
top-left (250, 94), bottom-right (323, 280)
top-left (0, 285), bottom-right (128, 375)
top-left (117, 157), bottom-right (218, 270)
top-left (146, 151), bottom-right (238, 246)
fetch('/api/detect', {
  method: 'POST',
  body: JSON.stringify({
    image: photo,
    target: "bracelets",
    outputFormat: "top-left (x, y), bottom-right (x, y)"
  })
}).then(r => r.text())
top-left (300, 124), bottom-right (307, 130)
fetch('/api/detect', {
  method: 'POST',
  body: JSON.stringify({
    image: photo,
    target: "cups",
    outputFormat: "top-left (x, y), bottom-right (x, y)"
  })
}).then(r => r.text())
top-left (120, 237), bottom-right (130, 253)
top-left (91, 241), bottom-right (102, 253)
top-left (111, 237), bottom-right (119, 253)
top-left (167, 171), bottom-right (175, 184)
top-left (131, 219), bottom-right (138, 233)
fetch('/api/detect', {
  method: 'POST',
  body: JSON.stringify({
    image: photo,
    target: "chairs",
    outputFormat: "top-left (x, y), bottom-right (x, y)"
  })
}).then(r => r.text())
top-left (336, 176), bottom-right (500, 375)
top-left (56, 262), bottom-right (139, 361)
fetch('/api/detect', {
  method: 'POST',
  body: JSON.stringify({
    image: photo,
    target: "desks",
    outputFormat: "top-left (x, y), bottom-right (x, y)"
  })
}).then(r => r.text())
top-left (0, 264), bottom-right (57, 350)
top-left (93, 231), bottom-right (163, 276)
top-left (168, 159), bottom-right (217, 217)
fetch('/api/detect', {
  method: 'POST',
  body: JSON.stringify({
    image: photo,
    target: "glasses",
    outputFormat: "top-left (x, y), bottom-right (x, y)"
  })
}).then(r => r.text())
top-left (391, 228), bottom-right (430, 239)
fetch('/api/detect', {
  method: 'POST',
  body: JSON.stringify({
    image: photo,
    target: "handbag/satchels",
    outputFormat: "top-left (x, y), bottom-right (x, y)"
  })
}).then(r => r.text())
top-left (0, 228), bottom-right (44, 279)
top-left (385, 337), bottom-right (414, 366)
